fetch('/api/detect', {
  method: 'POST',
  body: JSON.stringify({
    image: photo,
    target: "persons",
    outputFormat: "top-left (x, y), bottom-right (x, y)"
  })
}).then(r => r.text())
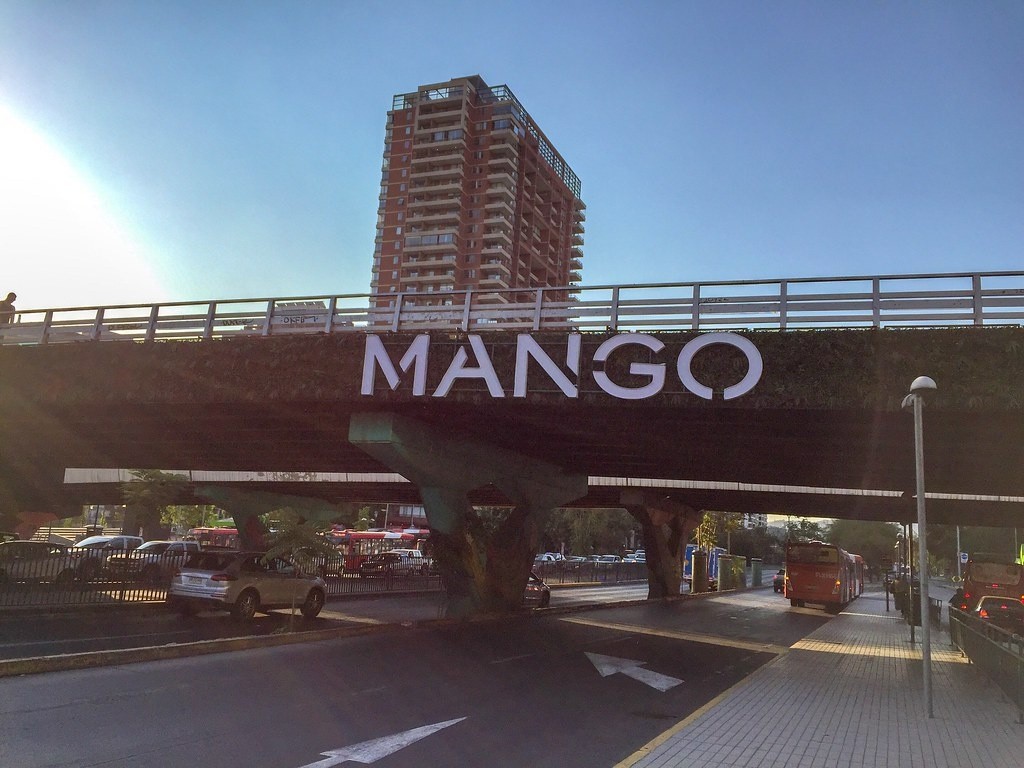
top-left (0, 292), bottom-right (17, 339)
top-left (948, 587), bottom-right (967, 608)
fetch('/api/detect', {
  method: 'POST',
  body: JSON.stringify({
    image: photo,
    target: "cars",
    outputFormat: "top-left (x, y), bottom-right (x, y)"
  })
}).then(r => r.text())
top-left (970, 596), bottom-right (1024, 636)
top-left (0, 526), bottom-right (431, 592)
top-left (532, 548), bottom-right (646, 578)
top-left (773, 569), bottom-right (785, 592)
top-left (523, 571), bottom-right (551, 610)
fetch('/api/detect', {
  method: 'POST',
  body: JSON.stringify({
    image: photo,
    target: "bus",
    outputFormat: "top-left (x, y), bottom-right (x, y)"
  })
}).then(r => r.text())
top-left (784, 541), bottom-right (865, 612)
top-left (962, 559), bottom-right (1024, 612)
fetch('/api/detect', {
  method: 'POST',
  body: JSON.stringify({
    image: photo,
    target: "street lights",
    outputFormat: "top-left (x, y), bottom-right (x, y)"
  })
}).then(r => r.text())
top-left (897, 532), bottom-right (903, 579)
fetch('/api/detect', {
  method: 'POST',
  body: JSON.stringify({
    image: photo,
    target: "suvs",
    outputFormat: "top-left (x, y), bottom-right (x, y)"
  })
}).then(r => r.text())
top-left (166, 549), bottom-right (328, 626)
